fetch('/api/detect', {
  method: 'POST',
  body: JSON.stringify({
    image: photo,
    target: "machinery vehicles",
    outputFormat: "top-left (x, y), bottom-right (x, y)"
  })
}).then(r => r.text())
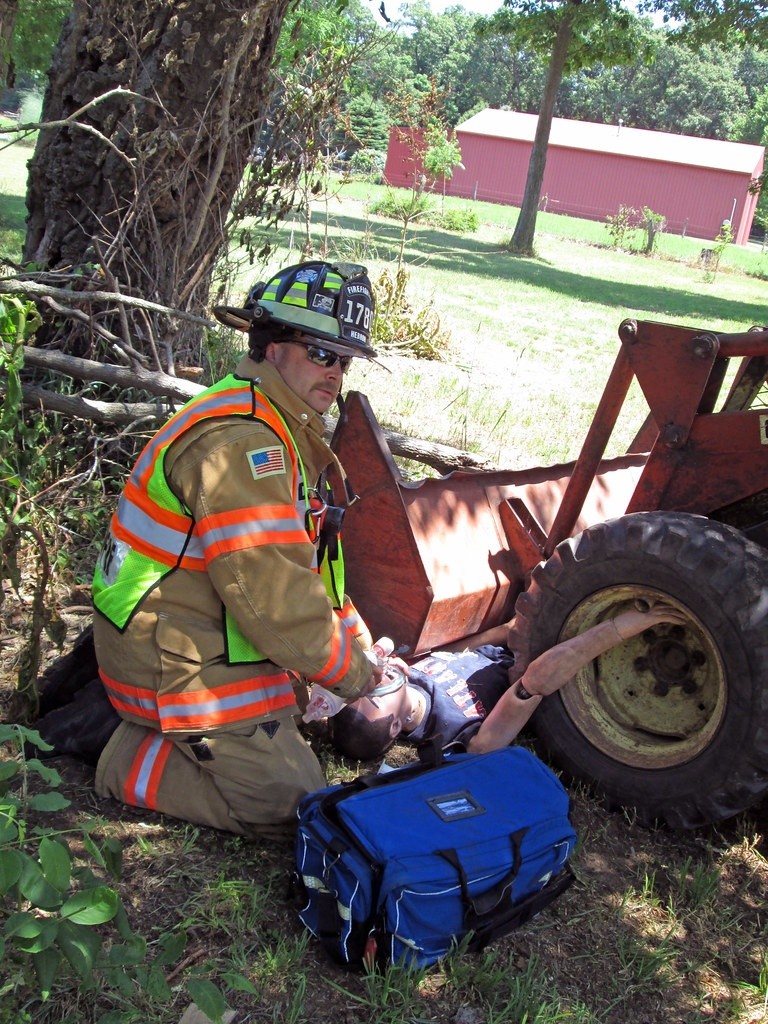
top-left (330, 317), bottom-right (767, 830)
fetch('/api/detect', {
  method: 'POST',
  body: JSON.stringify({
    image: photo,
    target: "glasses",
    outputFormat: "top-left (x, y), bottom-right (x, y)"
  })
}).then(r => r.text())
top-left (274, 339), bottom-right (353, 374)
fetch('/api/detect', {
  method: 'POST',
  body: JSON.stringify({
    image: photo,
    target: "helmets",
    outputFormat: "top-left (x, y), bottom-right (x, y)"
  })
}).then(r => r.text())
top-left (214, 261), bottom-right (378, 358)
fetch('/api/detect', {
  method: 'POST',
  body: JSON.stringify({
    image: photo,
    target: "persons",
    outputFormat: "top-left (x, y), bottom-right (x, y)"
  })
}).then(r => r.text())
top-left (20, 263), bottom-right (409, 837)
top-left (328, 605), bottom-right (689, 767)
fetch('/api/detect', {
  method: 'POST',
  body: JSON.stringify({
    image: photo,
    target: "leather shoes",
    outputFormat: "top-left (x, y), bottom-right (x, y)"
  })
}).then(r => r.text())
top-left (37, 623), bottom-right (98, 719)
top-left (24, 678), bottom-right (121, 769)
